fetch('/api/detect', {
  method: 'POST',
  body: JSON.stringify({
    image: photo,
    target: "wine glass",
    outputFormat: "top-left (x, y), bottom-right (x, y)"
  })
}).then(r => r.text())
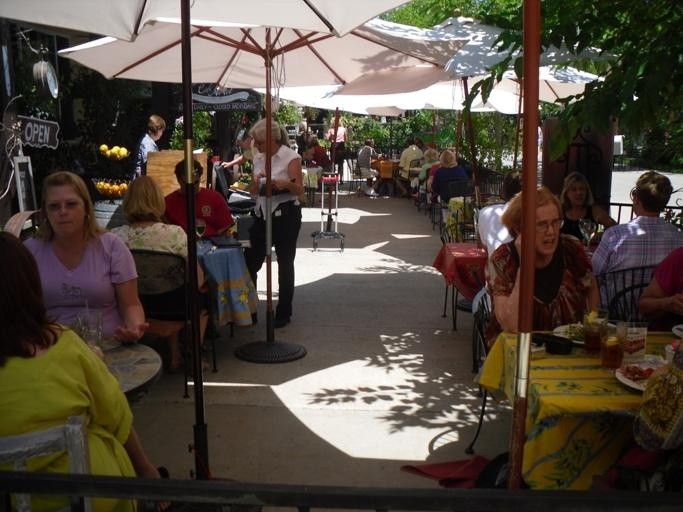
top-left (584, 222), bottom-right (596, 247)
top-left (196, 217), bottom-right (207, 241)
top-left (580, 218), bottom-right (591, 238)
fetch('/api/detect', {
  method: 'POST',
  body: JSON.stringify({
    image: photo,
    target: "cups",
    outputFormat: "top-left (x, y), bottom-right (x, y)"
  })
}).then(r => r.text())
top-left (583, 310), bottom-right (647, 369)
top-left (77, 310), bottom-right (102, 347)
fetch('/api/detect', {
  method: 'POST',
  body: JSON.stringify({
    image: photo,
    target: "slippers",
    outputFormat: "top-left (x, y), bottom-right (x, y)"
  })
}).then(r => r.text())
top-left (399, 191), bottom-right (409, 197)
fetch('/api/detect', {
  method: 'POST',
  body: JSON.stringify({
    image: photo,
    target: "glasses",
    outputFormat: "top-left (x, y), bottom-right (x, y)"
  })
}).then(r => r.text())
top-left (533, 218), bottom-right (564, 233)
top-left (632, 190), bottom-right (637, 197)
top-left (178, 178), bottom-right (199, 186)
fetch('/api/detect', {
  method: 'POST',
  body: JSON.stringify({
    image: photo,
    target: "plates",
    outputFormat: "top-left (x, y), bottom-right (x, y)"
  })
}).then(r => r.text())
top-left (615, 363), bottom-right (662, 393)
top-left (672, 325), bottom-right (683, 338)
top-left (552, 323), bottom-right (624, 348)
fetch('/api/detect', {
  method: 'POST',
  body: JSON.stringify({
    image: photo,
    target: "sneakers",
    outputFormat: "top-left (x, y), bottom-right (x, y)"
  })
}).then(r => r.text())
top-left (340, 179), bottom-right (343, 183)
top-left (363, 185), bottom-right (380, 196)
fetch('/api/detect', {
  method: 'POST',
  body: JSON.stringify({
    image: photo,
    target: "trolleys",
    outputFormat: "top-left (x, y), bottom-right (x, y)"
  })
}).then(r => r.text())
top-left (310, 170), bottom-right (345, 248)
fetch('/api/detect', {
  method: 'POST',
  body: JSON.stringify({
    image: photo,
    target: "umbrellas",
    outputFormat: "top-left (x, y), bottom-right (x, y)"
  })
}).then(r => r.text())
top-left (0, 2), bottom-right (411, 478)
top-left (55, 19), bottom-right (471, 343)
top-left (439, 66), bottom-right (638, 170)
top-left (322, 8), bottom-right (627, 206)
top-left (251, 84), bottom-right (407, 175)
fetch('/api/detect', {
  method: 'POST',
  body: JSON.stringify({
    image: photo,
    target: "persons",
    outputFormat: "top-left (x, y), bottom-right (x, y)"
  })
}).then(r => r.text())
top-left (1, 232), bottom-right (175, 511)
top-left (324, 116), bottom-right (348, 184)
top-left (107, 114), bottom-right (330, 369)
top-left (356, 136), bottom-right (383, 198)
top-left (24, 170), bottom-right (151, 348)
top-left (392, 135), bottom-right (682, 493)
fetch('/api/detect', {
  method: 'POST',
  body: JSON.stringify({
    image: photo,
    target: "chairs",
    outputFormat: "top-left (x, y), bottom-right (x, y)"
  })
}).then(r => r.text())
top-left (127, 249), bottom-right (218, 398)
top-left (343, 147), bottom-right (373, 197)
top-left (0, 417), bottom-right (91, 512)
top-left (237, 217), bottom-right (266, 290)
top-left (466, 287), bottom-right (491, 397)
top-left (215, 165), bottom-right (256, 215)
top-left (609, 450), bottom-right (683, 492)
top-left (430, 177), bottom-right (470, 235)
top-left (400, 158), bottom-right (431, 216)
top-left (448, 180), bottom-right (476, 241)
top-left (607, 284), bottom-right (672, 331)
top-left (440, 221), bottom-right (475, 244)
top-left (596, 264), bottom-right (662, 320)
